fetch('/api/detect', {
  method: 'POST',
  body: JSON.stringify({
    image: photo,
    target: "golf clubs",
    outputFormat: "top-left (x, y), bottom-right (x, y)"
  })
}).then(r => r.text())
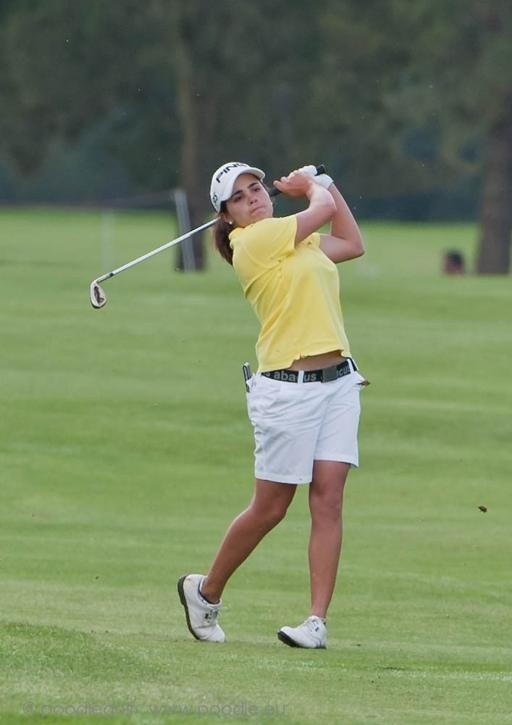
top-left (91, 163), bottom-right (327, 309)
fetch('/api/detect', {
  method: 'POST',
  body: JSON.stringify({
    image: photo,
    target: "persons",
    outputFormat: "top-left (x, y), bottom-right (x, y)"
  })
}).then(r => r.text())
top-left (177, 161), bottom-right (367, 650)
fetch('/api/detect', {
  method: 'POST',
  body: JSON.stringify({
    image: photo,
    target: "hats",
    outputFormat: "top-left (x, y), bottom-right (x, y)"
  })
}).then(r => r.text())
top-left (209, 162), bottom-right (265, 214)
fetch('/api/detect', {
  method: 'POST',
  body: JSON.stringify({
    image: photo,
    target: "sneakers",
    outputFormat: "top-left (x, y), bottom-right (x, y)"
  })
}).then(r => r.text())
top-left (177, 573), bottom-right (225, 643)
top-left (278, 615), bottom-right (327, 649)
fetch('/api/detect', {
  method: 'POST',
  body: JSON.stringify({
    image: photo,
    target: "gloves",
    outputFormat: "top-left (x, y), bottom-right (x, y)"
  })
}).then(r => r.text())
top-left (298, 165), bottom-right (334, 190)
